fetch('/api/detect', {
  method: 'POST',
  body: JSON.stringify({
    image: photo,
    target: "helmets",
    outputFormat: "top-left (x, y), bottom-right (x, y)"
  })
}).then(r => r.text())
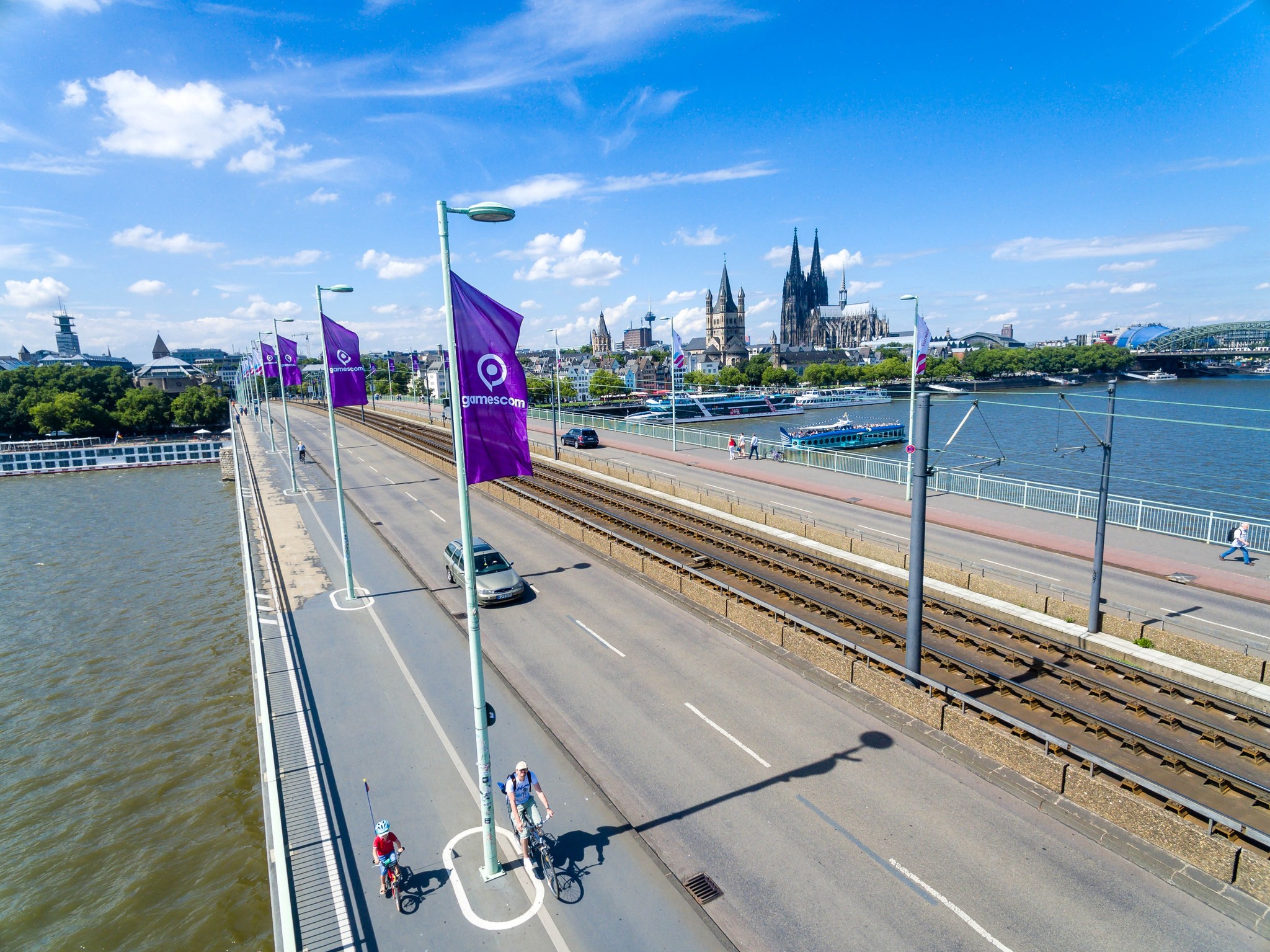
top-left (375, 820), bottom-right (390, 834)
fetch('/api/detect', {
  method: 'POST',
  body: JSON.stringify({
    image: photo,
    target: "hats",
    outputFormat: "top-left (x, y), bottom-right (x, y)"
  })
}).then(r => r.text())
top-left (378, 830), bottom-right (389, 837)
top-left (516, 761), bottom-right (528, 770)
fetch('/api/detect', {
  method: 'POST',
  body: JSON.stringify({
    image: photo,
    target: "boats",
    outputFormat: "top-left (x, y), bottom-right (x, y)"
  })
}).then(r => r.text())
top-left (780, 412), bottom-right (907, 452)
top-left (792, 385), bottom-right (892, 409)
top-left (1255, 363), bottom-right (1270, 374)
top-left (623, 389), bottom-right (804, 427)
top-left (1142, 366), bottom-right (1178, 382)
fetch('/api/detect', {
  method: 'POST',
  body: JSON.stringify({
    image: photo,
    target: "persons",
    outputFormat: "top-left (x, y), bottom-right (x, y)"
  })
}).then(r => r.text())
top-left (232, 400), bottom-right (258, 423)
top-left (375, 393), bottom-right (425, 405)
top-left (728, 433), bottom-right (759, 460)
top-left (0, 443), bottom-right (83, 453)
top-left (505, 760), bottom-right (554, 872)
top-left (851, 422), bottom-right (901, 429)
top-left (555, 387), bottom-right (800, 410)
top-left (1219, 523), bottom-right (1255, 565)
top-left (199, 434), bottom-right (224, 442)
top-left (788, 428), bottom-right (832, 438)
top-left (486, 554), bottom-right (497, 566)
top-left (801, 370), bottom-right (1047, 398)
top-left (372, 820), bottom-right (403, 895)
top-left (296, 441), bottom-right (306, 460)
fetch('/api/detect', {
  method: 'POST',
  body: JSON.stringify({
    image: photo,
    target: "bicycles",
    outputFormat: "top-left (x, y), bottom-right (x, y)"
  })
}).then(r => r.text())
top-left (520, 809), bottom-right (560, 899)
top-left (765, 446), bottom-right (784, 463)
top-left (371, 847), bottom-right (406, 912)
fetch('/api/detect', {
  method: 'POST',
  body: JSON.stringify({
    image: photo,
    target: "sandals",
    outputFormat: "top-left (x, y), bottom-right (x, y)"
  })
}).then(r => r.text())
top-left (394, 864), bottom-right (401, 877)
top-left (380, 884), bottom-right (386, 895)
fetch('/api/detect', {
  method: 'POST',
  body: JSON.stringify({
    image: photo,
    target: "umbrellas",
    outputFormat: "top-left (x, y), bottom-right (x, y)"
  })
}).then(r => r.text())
top-left (194, 428), bottom-right (232, 437)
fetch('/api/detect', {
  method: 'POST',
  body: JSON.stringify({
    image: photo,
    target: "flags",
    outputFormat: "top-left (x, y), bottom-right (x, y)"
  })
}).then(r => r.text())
top-left (673, 328), bottom-right (685, 369)
top-left (370, 355), bottom-right (418, 374)
top-left (444, 270), bottom-right (533, 486)
top-left (118, 434), bottom-right (123, 439)
top-left (556, 338), bottom-right (561, 368)
top-left (234, 334), bottom-right (303, 408)
top-left (318, 311), bottom-right (369, 410)
top-left (915, 314), bottom-right (932, 375)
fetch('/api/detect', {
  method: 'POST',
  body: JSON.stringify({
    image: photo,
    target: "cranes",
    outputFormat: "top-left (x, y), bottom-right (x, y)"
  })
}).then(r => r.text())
top-left (291, 331), bottom-right (321, 359)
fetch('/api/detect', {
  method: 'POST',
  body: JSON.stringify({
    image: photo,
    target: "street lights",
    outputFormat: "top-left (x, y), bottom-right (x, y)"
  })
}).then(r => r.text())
top-left (436, 343), bottom-right (447, 399)
top-left (383, 354), bottom-right (393, 403)
top-left (545, 328), bottom-right (562, 429)
top-left (229, 379), bottom-right (232, 400)
top-left (364, 358), bottom-right (373, 395)
top-left (233, 347), bottom-right (257, 421)
top-left (660, 316), bottom-right (677, 451)
top-left (251, 340), bottom-right (263, 433)
top-left (405, 348), bottom-right (417, 405)
top-left (314, 283), bottom-right (358, 601)
top-left (273, 317), bottom-right (298, 492)
top-left (900, 293), bottom-right (919, 500)
top-left (258, 331), bottom-right (276, 452)
top-left (433, 196), bottom-right (516, 883)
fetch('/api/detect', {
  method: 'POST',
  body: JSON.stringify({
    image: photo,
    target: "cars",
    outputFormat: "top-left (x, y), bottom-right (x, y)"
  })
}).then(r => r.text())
top-left (442, 536), bottom-right (525, 608)
top-left (247, 392), bottom-right (324, 405)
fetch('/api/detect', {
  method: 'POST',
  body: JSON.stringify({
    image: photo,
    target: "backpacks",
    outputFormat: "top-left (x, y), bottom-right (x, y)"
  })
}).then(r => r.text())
top-left (502, 771), bottom-right (532, 795)
top-left (1227, 527), bottom-right (1242, 542)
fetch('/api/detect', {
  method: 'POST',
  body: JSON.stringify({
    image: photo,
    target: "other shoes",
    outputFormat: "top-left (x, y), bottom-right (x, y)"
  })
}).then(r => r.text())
top-left (729, 458), bottom-right (732, 460)
top-left (1219, 555), bottom-right (1225, 561)
top-left (747, 457), bottom-right (750, 459)
top-left (738, 456), bottom-right (741, 457)
top-left (742, 456), bottom-right (745, 457)
top-left (755, 459), bottom-right (758, 460)
top-left (523, 858), bottom-right (533, 871)
top-left (733, 456), bottom-right (735, 459)
top-left (1248, 563), bottom-right (1255, 565)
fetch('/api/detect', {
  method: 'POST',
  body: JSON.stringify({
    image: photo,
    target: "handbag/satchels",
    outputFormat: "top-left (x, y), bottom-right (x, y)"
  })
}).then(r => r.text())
top-left (734, 441), bottom-right (736, 446)
top-left (737, 446), bottom-right (741, 451)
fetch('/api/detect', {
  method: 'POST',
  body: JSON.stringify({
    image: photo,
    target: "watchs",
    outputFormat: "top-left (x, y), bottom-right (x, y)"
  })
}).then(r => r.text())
top-left (546, 806), bottom-right (551, 809)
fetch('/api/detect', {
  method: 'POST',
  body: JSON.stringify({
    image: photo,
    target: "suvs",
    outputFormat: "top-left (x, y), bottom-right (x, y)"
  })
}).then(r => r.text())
top-left (560, 427), bottom-right (599, 449)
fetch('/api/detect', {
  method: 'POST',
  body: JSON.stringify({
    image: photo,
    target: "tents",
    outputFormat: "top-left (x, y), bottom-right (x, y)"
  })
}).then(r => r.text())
top-left (601, 382), bottom-right (797, 400)
